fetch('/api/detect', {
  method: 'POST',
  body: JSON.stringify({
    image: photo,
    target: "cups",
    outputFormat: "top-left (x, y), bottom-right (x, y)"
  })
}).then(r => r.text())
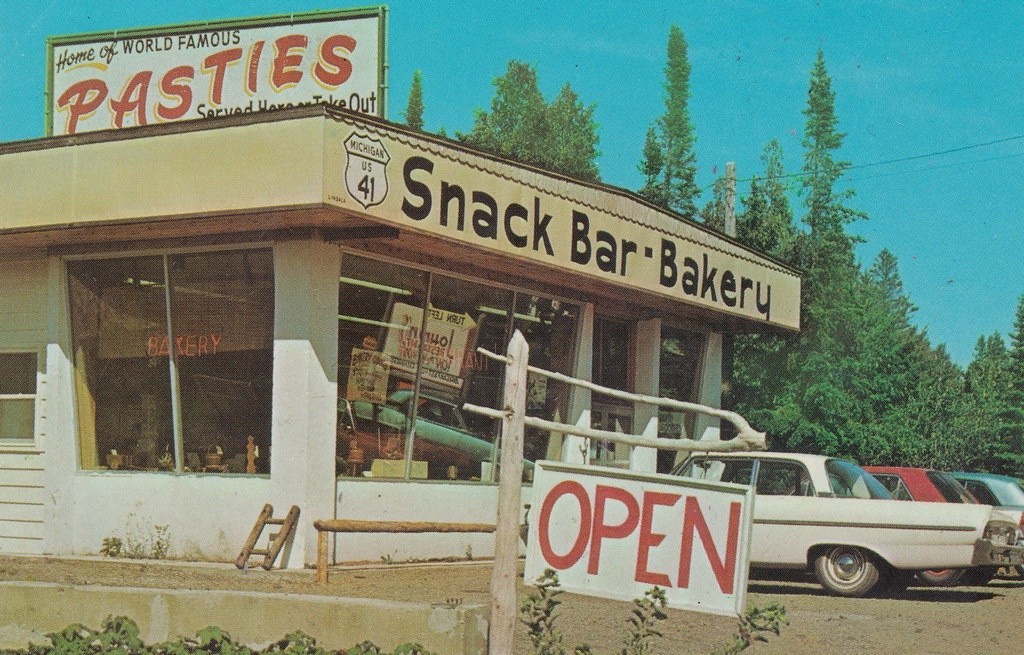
top-left (446, 465), bottom-right (458, 480)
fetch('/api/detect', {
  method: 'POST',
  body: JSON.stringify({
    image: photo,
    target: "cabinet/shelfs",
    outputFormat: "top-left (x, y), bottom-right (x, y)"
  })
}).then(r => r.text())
top-left (591, 402), bottom-right (634, 472)
top-left (350, 346), bottom-right (391, 405)
top-left (657, 410), bottom-right (693, 478)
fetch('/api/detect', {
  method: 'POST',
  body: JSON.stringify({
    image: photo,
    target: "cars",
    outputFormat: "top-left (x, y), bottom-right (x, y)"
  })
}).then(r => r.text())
top-left (893, 470), bottom-right (1024, 506)
top-left (334, 385), bottom-right (533, 483)
top-left (787, 464), bottom-right (981, 586)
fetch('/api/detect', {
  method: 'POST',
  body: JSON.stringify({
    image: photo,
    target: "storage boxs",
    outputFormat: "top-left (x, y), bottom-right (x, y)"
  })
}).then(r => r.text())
top-left (382, 302), bottom-right (482, 397)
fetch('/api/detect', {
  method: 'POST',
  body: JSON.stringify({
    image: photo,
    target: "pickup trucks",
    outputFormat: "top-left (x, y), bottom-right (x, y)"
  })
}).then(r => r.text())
top-left (668, 450), bottom-right (1024, 599)
